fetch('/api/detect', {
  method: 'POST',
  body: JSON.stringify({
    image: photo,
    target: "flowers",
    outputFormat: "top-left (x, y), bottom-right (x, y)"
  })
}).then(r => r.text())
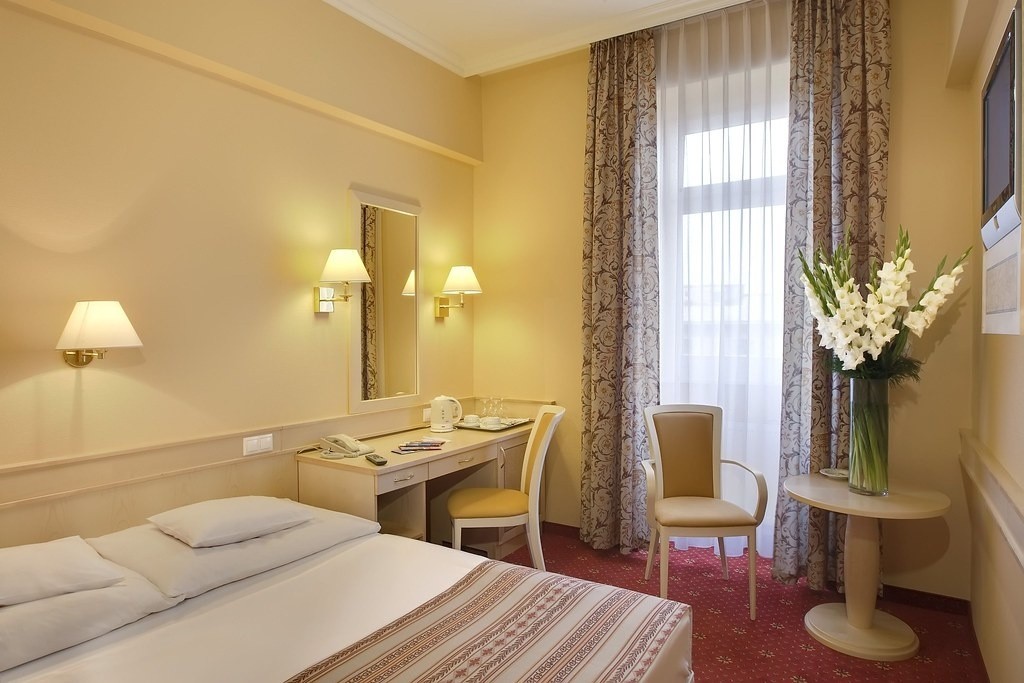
top-left (797, 220), bottom-right (975, 392)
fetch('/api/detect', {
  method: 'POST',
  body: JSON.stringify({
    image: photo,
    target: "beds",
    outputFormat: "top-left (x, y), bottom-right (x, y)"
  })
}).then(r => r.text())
top-left (0, 532), bottom-right (696, 683)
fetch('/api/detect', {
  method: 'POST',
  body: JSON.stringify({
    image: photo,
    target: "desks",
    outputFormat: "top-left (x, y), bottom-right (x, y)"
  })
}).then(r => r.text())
top-left (783, 474), bottom-right (952, 662)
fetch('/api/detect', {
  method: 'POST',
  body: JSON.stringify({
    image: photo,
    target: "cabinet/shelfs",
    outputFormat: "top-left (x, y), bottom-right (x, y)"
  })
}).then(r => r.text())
top-left (294, 415), bottom-right (545, 561)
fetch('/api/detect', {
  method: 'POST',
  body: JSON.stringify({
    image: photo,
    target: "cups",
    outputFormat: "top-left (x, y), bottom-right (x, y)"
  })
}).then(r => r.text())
top-left (464, 415), bottom-right (480, 426)
top-left (482, 417), bottom-right (500, 429)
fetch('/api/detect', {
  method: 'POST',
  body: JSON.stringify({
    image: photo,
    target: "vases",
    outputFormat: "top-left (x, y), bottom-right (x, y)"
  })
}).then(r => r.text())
top-left (846, 377), bottom-right (888, 498)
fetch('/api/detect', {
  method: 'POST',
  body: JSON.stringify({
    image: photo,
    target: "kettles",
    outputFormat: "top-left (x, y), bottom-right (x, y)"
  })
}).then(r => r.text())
top-left (430, 395), bottom-right (463, 432)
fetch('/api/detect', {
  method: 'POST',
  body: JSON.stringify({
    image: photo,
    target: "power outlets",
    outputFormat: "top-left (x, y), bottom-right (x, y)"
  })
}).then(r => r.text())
top-left (423, 408), bottom-right (432, 422)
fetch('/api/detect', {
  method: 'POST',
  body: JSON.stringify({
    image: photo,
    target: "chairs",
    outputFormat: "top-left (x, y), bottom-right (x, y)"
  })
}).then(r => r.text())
top-left (642, 404), bottom-right (768, 620)
top-left (447, 405), bottom-right (566, 571)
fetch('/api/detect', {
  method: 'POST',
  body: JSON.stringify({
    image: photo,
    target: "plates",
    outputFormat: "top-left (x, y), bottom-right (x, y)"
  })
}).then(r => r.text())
top-left (820, 469), bottom-right (849, 480)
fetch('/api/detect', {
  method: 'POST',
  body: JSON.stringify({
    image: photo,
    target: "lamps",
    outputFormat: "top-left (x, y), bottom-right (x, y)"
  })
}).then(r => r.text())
top-left (313, 249), bottom-right (372, 313)
top-left (434, 265), bottom-right (483, 317)
top-left (54, 300), bottom-right (143, 368)
top-left (402, 269), bottom-right (415, 296)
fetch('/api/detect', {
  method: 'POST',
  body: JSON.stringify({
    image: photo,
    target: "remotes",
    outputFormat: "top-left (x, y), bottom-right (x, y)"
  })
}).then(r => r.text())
top-left (365, 453), bottom-right (388, 466)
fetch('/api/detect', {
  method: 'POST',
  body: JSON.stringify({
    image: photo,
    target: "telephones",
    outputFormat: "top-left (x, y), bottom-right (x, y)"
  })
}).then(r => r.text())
top-left (319, 433), bottom-right (376, 457)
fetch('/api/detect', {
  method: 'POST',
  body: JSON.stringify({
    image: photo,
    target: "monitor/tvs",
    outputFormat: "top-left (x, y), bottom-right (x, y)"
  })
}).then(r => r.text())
top-left (981, 7), bottom-right (1015, 228)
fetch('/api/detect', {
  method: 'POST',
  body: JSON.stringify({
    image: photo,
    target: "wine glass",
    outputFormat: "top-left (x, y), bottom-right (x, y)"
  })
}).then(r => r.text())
top-left (480, 397), bottom-right (508, 421)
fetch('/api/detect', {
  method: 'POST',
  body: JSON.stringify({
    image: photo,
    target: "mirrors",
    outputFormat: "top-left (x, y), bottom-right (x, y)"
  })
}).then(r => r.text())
top-left (348, 189), bottom-right (423, 415)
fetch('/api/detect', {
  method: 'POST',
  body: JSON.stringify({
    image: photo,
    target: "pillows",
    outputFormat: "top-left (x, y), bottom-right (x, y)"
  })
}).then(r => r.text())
top-left (85, 499), bottom-right (382, 598)
top-left (145, 496), bottom-right (316, 548)
top-left (0, 558), bottom-right (186, 673)
top-left (0, 535), bottom-right (126, 607)
